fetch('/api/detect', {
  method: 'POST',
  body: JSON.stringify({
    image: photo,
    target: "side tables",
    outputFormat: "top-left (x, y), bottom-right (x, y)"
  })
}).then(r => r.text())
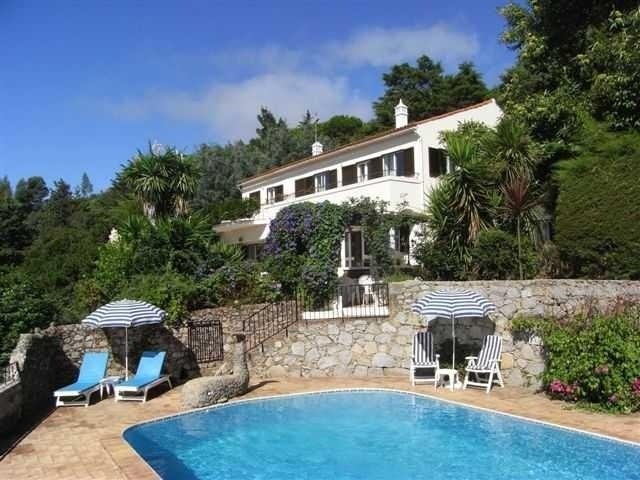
top-left (435, 369), bottom-right (460, 393)
top-left (99, 376), bottom-right (124, 400)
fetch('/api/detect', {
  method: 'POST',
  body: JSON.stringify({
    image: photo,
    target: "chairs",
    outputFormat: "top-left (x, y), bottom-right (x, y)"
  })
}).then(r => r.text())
top-left (409, 331), bottom-right (441, 386)
top-left (358, 275), bottom-right (375, 305)
top-left (114, 350), bottom-right (173, 403)
top-left (53, 352), bottom-right (109, 408)
top-left (463, 335), bottom-right (504, 393)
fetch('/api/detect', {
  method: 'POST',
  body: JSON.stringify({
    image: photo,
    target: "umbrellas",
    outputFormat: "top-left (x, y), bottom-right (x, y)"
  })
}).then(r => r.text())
top-left (409, 287), bottom-right (497, 369)
top-left (81, 298), bottom-right (171, 383)
